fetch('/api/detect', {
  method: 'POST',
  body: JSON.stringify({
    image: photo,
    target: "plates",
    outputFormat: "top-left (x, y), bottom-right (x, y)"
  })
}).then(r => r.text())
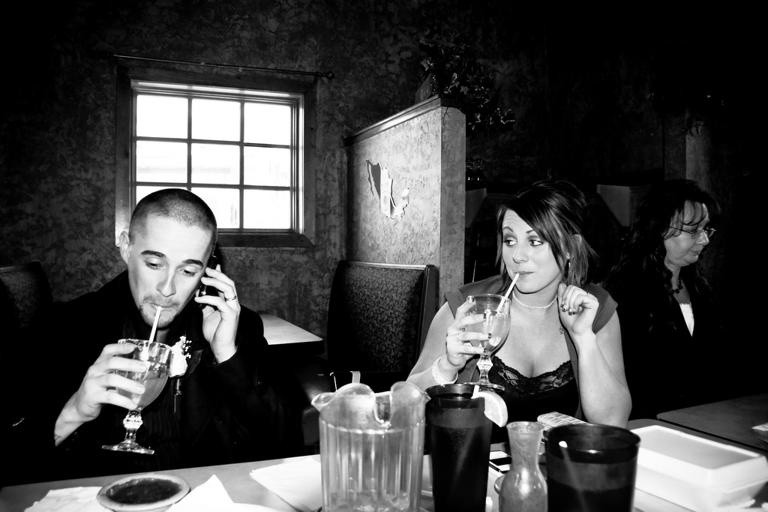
top-left (420, 451), bottom-right (432, 497)
top-left (751, 423), bottom-right (768, 443)
top-left (96, 473), bottom-right (191, 510)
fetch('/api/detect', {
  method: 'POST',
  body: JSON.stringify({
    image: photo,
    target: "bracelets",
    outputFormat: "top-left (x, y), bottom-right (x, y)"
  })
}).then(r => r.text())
top-left (430, 356), bottom-right (459, 385)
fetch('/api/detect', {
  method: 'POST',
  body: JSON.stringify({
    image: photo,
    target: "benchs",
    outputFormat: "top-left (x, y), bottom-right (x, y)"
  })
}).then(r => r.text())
top-left (302, 258), bottom-right (437, 444)
top-left (0, 260), bottom-right (54, 336)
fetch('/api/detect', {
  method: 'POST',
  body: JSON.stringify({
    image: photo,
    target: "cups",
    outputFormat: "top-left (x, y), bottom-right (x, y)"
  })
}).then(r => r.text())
top-left (320, 393), bottom-right (425, 512)
top-left (544, 424), bottom-right (641, 512)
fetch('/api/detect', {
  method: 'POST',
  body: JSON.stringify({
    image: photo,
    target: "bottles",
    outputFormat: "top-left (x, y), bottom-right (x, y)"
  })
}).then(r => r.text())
top-left (426, 384), bottom-right (505, 512)
top-left (498, 420), bottom-right (547, 512)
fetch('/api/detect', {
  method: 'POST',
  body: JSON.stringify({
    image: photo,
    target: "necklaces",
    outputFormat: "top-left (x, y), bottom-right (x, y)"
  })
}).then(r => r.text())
top-left (512, 291), bottom-right (557, 310)
top-left (671, 277), bottom-right (683, 294)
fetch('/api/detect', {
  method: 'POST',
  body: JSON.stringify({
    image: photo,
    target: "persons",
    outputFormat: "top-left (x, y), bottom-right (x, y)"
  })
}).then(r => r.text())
top-left (404, 178), bottom-right (633, 454)
top-left (603, 178), bottom-right (722, 421)
top-left (1, 189), bottom-right (303, 491)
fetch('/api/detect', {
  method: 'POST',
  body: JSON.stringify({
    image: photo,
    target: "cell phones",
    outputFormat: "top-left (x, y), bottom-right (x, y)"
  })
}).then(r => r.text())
top-left (199, 245), bottom-right (222, 310)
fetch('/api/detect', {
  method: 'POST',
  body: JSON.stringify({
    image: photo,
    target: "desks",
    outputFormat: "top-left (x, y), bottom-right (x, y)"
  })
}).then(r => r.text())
top-left (262, 309), bottom-right (324, 355)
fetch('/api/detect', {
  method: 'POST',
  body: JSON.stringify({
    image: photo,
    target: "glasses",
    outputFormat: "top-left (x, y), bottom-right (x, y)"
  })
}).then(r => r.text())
top-left (668, 224), bottom-right (716, 239)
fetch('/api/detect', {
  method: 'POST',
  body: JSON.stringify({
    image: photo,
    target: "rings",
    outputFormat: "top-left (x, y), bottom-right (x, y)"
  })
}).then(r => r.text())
top-left (225, 297), bottom-right (237, 303)
top-left (586, 291), bottom-right (589, 295)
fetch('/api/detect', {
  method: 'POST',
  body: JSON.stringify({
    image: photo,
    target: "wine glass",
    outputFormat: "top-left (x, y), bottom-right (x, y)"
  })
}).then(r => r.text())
top-left (463, 293), bottom-right (512, 390)
top-left (102, 339), bottom-right (174, 455)
